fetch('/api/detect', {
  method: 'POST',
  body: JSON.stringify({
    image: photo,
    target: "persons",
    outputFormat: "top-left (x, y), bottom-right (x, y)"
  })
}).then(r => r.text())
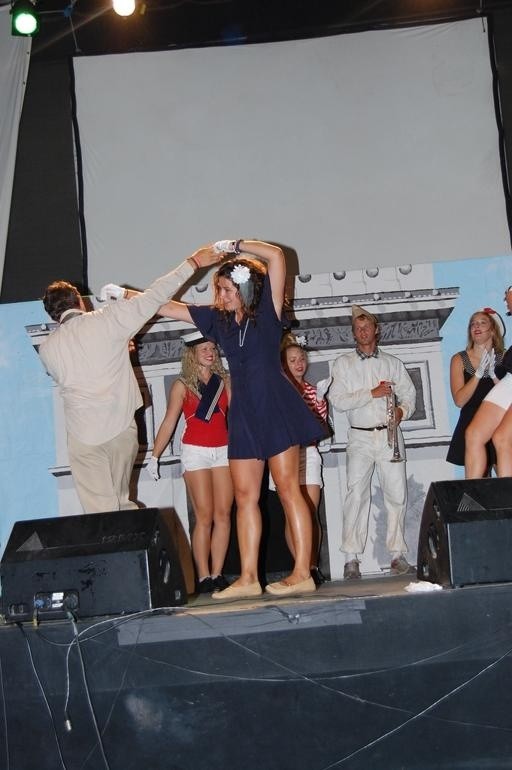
top-left (327, 303), bottom-right (419, 583)
top-left (96, 239), bottom-right (327, 601)
top-left (444, 307), bottom-right (506, 477)
top-left (266, 332), bottom-right (336, 589)
top-left (462, 371), bottom-right (512, 481)
top-left (37, 246), bottom-right (228, 515)
top-left (145, 331), bottom-right (232, 594)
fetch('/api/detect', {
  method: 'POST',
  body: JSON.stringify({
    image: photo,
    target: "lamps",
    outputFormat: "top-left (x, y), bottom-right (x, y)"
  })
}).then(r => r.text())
top-left (8, 0), bottom-right (40, 38)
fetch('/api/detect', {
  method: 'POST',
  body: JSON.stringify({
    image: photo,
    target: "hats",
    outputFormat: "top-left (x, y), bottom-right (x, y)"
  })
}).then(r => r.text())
top-left (180, 331), bottom-right (208, 347)
top-left (351, 304), bottom-right (370, 323)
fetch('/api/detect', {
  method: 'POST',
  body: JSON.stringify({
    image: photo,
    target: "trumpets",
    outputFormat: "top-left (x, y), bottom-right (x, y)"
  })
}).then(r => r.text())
top-left (386, 380), bottom-right (405, 463)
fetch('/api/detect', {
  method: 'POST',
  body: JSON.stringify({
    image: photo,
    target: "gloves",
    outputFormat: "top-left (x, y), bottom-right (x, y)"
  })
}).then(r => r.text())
top-left (316, 375), bottom-right (333, 401)
top-left (99, 283), bottom-right (125, 303)
top-left (213, 240), bottom-right (237, 254)
top-left (474, 348), bottom-right (491, 378)
top-left (487, 348), bottom-right (496, 379)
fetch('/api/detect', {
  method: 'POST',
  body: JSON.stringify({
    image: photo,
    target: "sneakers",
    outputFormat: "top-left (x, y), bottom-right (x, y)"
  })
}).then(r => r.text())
top-left (212, 575), bottom-right (231, 591)
top-left (265, 576), bottom-right (317, 596)
top-left (197, 577), bottom-right (220, 595)
top-left (344, 562), bottom-right (361, 580)
top-left (390, 556), bottom-right (416, 577)
top-left (310, 568), bottom-right (325, 584)
top-left (211, 579), bottom-right (262, 600)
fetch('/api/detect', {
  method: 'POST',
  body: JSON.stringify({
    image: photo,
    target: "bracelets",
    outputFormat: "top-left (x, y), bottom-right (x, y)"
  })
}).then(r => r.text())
top-left (124, 288), bottom-right (129, 299)
top-left (235, 239), bottom-right (245, 253)
top-left (189, 256), bottom-right (202, 271)
